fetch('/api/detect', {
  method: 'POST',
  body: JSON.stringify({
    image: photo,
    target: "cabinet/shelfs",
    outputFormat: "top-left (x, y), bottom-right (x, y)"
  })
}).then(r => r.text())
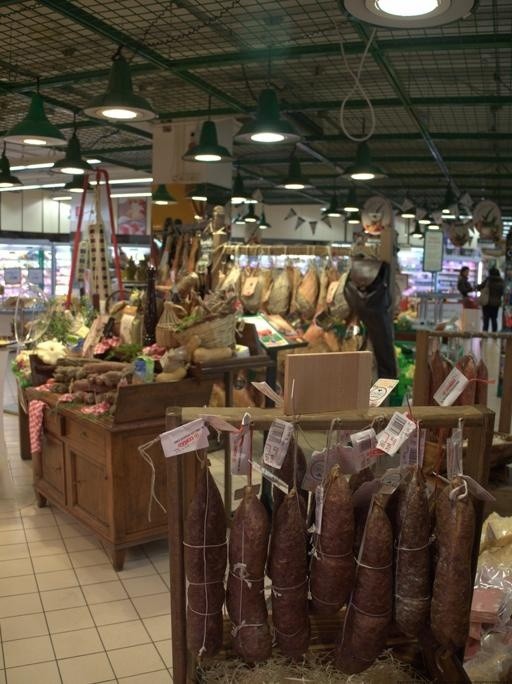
top-left (1, 230), bottom-right (503, 339)
top-left (28, 387), bottom-right (178, 571)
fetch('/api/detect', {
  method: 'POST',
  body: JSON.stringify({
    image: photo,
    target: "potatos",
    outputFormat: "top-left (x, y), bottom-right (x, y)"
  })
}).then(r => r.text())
top-left (49, 358), bottom-right (135, 400)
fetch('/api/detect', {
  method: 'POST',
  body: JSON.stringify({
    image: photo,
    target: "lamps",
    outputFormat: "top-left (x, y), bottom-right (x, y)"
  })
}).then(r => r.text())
top-left (0, 0), bottom-right (512, 239)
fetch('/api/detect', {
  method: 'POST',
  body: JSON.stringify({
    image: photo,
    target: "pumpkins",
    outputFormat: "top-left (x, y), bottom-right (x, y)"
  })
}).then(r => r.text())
top-left (154, 333), bottom-right (234, 384)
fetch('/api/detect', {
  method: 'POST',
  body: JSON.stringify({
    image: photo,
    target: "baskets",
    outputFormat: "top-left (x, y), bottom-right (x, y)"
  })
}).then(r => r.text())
top-left (106, 290), bottom-right (134, 337)
top-left (173, 296), bottom-right (235, 348)
top-left (156, 326), bottom-right (181, 347)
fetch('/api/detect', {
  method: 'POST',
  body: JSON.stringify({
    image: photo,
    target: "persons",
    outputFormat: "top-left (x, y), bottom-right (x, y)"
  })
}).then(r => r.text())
top-left (476, 267), bottom-right (507, 332)
top-left (456, 264), bottom-right (474, 298)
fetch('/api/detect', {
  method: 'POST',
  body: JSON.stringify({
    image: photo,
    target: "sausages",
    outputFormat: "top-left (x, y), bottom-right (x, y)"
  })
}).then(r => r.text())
top-left (183, 434), bottom-right (474, 684)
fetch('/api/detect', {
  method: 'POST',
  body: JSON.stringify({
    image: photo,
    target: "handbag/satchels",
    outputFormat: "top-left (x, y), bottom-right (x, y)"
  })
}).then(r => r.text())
top-left (220, 258), bottom-right (389, 319)
top-left (480, 287), bottom-right (489, 305)
